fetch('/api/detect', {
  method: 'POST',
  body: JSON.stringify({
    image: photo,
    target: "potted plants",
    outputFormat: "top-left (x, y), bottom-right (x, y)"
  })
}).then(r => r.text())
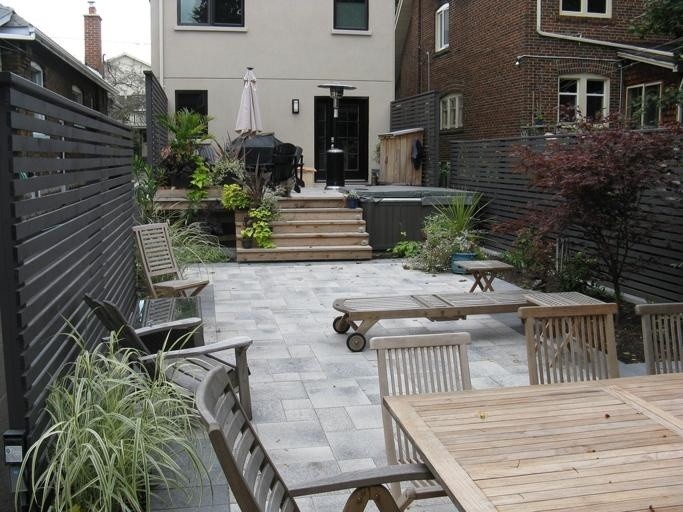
top-left (428, 223), bottom-right (486, 276)
top-left (238, 201), bottom-right (281, 249)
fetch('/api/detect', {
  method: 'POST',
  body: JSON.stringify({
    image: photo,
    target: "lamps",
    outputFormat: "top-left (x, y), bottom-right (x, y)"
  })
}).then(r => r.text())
top-left (291, 98), bottom-right (299, 114)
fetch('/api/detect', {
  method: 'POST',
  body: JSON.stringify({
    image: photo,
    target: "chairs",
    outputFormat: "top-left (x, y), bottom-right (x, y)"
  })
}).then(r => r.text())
top-left (263, 142), bottom-right (305, 196)
top-left (365, 330), bottom-right (475, 507)
top-left (197, 362), bottom-right (449, 512)
top-left (83, 292), bottom-right (256, 423)
top-left (635, 301), bottom-right (681, 373)
top-left (129, 221), bottom-right (211, 300)
top-left (515, 302), bottom-right (621, 386)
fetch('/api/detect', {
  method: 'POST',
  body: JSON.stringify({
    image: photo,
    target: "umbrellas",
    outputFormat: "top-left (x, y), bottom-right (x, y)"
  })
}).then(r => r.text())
top-left (234, 71), bottom-right (265, 136)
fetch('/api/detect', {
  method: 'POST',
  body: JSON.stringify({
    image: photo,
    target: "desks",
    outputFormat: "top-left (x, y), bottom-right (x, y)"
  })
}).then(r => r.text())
top-left (127, 298), bottom-right (204, 352)
top-left (454, 259), bottom-right (514, 290)
top-left (378, 372), bottom-right (682, 508)
